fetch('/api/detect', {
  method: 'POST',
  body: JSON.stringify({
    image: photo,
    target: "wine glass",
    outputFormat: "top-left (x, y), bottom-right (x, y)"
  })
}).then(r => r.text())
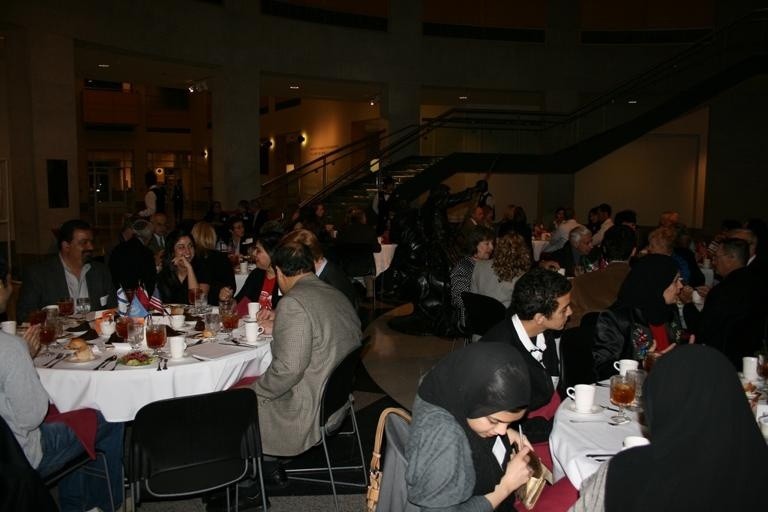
top-left (608, 374), bottom-right (635, 423)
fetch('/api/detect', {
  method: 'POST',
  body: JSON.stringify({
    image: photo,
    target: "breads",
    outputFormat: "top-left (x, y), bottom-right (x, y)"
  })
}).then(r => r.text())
top-left (70, 338), bottom-right (87, 350)
top-left (74, 346), bottom-right (95, 360)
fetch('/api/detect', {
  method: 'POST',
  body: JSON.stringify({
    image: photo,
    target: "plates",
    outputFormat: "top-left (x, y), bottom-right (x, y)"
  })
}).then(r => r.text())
top-left (563, 402), bottom-right (606, 415)
top-left (17, 302), bottom-right (265, 368)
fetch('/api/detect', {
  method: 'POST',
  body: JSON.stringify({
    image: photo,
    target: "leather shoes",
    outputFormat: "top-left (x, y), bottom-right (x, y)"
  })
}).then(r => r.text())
top-left (204, 486), bottom-right (271, 512)
top-left (258, 466), bottom-right (289, 491)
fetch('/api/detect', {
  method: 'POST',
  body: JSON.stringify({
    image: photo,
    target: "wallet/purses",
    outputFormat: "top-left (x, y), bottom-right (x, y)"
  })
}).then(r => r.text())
top-left (502, 440), bottom-right (553, 510)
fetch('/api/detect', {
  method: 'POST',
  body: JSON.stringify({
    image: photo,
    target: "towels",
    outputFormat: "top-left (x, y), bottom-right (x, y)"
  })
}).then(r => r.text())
top-left (512, 391), bottom-right (580, 512)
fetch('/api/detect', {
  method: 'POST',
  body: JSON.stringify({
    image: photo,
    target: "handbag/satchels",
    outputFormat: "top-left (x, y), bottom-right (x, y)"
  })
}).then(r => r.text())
top-left (366, 471), bottom-right (383, 511)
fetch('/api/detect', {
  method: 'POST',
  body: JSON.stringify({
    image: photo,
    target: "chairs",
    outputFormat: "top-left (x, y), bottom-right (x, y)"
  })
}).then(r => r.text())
top-left (464, 292), bottom-right (508, 349)
top-left (560, 326), bottom-right (588, 386)
top-left (384, 413), bottom-right (411, 468)
top-left (0, 388), bottom-right (271, 512)
top-left (279, 336), bottom-right (374, 512)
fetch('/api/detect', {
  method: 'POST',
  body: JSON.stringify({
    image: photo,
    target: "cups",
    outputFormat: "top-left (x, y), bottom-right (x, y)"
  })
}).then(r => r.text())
top-left (735, 356), bottom-right (768, 438)
top-left (704, 258), bottom-right (711, 269)
top-left (612, 359), bottom-right (638, 376)
top-left (626, 368), bottom-right (646, 412)
top-left (642, 351), bottom-right (662, 371)
top-left (552, 260), bottom-right (606, 278)
top-left (621, 436), bottom-right (649, 449)
top-left (567, 384), bottom-right (595, 411)
top-left (2, 321), bottom-right (17, 336)
